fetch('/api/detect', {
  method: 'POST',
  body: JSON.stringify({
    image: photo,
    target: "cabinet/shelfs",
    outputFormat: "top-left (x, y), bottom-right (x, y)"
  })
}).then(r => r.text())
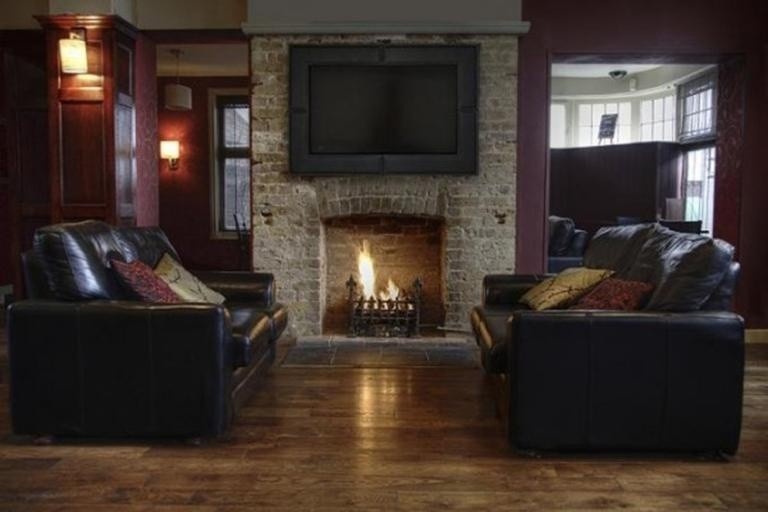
top-left (550, 141), bottom-right (686, 222)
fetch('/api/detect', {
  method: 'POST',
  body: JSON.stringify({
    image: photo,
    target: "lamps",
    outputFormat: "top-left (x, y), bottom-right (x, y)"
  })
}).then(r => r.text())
top-left (159, 140), bottom-right (180, 169)
top-left (165, 48), bottom-right (193, 111)
top-left (58, 31), bottom-right (88, 74)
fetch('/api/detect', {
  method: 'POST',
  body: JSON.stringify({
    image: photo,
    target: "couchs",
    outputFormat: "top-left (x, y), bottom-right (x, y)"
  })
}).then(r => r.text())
top-left (6, 219), bottom-right (287, 439)
top-left (470, 260), bottom-right (745, 455)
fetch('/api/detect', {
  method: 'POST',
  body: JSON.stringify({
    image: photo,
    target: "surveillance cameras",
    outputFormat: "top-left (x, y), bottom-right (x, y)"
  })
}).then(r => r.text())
top-left (608, 69), bottom-right (628, 81)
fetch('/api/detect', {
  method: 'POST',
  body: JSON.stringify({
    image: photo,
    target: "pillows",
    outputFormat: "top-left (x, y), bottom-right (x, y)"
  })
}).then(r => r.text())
top-left (517, 214), bottom-right (735, 315)
top-left (152, 251), bottom-right (226, 305)
top-left (38, 229), bottom-right (124, 302)
top-left (109, 258), bottom-right (180, 304)
top-left (123, 226), bottom-right (177, 269)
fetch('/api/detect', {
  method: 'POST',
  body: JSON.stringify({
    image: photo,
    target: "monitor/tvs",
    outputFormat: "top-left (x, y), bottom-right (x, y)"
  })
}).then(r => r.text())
top-left (289, 42), bottom-right (479, 176)
